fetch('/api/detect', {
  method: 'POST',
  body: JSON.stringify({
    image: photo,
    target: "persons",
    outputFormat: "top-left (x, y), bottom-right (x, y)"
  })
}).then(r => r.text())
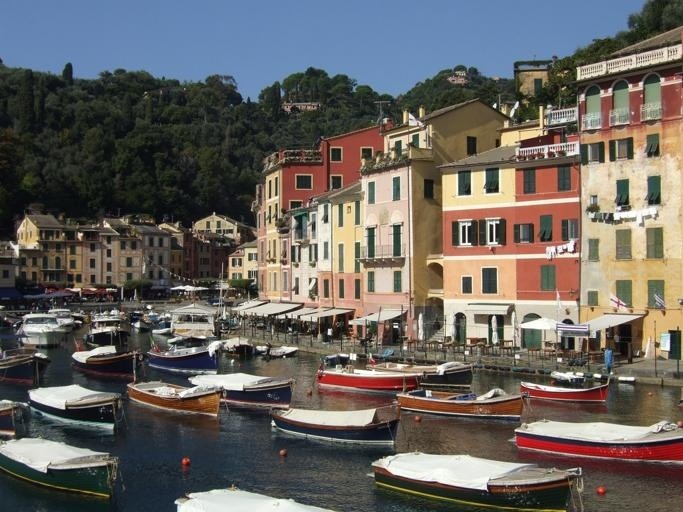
top-left (35, 303), bottom-right (39, 313)
top-left (604, 346), bottom-right (614, 373)
top-left (359, 330), bottom-right (373, 346)
top-left (32, 303), bottom-right (36, 313)
top-left (327, 325), bottom-right (334, 343)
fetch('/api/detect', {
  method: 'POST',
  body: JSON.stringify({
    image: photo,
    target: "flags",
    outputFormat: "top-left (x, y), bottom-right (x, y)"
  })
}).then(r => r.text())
top-left (654, 290), bottom-right (665, 309)
top-left (610, 293), bottom-right (627, 311)
top-left (556, 290), bottom-right (562, 309)
top-left (406, 111), bottom-right (426, 129)
top-left (644, 336), bottom-right (652, 360)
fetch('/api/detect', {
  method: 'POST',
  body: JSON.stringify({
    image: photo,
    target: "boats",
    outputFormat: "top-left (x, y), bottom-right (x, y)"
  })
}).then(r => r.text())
top-left (269, 402), bottom-right (402, 446)
top-left (0, 400), bottom-right (31, 439)
top-left (395, 388), bottom-right (527, 419)
top-left (188, 372), bottom-right (294, 410)
top-left (513, 417), bottom-right (682, 462)
top-left (325, 352), bottom-right (351, 367)
top-left (364, 361), bottom-right (473, 389)
top-left (520, 380), bottom-right (609, 402)
top-left (369, 451), bottom-right (581, 511)
top-left (173, 485), bottom-right (333, 512)
top-left (127, 379), bottom-right (224, 417)
top-left (551, 371), bottom-right (586, 386)
top-left (0, 437), bottom-right (118, 499)
top-left (27, 384), bottom-right (122, 431)
top-left (317, 366), bottom-right (424, 390)
top-left (2, 301), bottom-right (299, 386)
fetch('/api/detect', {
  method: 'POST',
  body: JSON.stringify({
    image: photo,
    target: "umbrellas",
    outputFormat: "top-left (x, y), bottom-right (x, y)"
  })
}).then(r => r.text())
top-left (169, 282), bottom-right (209, 293)
top-left (24, 286), bottom-right (119, 300)
top-left (519, 317), bottom-right (566, 347)
top-left (490, 315), bottom-right (500, 344)
top-left (416, 311), bottom-right (424, 341)
